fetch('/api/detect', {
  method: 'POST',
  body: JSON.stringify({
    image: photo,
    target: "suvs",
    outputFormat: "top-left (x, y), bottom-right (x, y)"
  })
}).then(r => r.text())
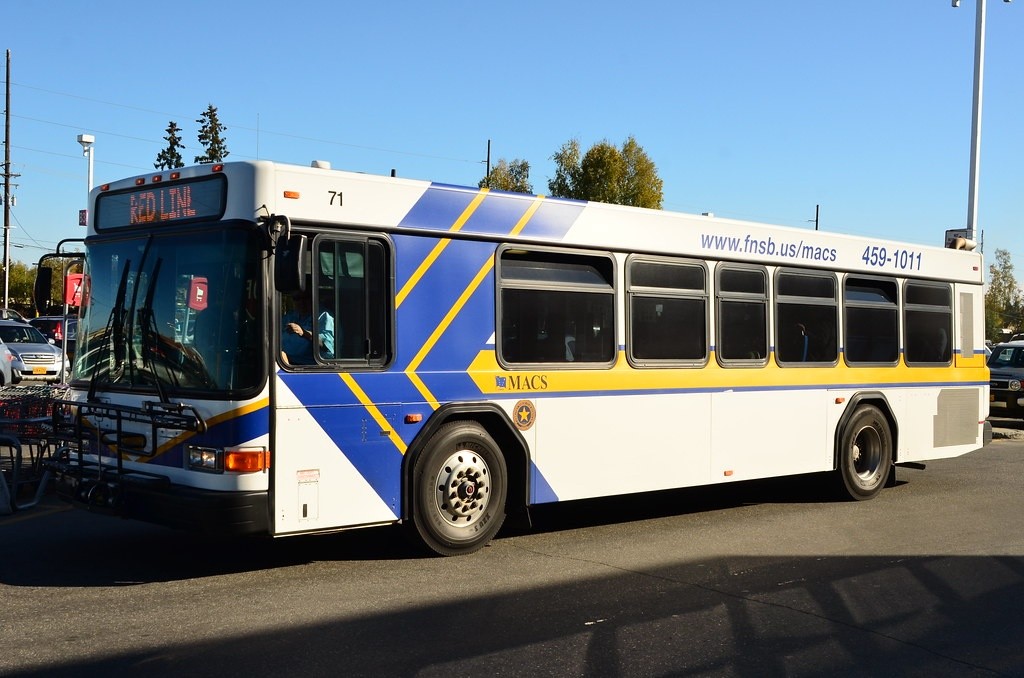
top-left (0, 319), bottom-right (71, 386)
top-left (28, 314), bottom-right (79, 349)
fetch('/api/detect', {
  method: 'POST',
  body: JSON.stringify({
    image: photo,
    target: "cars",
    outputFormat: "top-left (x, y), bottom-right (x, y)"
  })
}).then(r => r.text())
top-left (1000, 334), bottom-right (1024, 360)
top-left (987, 341), bottom-right (1024, 414)
top-left (1, 308), bottom-right (27, 322)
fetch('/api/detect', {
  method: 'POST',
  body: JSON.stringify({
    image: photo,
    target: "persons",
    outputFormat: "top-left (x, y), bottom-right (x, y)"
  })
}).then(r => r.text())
top-left (282, 284), bottom-right (334, 358)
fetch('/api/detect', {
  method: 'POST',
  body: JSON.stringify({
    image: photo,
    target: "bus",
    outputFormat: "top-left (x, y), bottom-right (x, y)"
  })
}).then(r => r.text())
top-left (33, 159), bottom-right (992, 557)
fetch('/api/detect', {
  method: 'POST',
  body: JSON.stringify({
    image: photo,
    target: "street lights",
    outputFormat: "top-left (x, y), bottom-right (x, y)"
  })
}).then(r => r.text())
top-left (3, 226), bottom-right (25, 308)
top-left (76, 133), bottom-right (94, 193)
